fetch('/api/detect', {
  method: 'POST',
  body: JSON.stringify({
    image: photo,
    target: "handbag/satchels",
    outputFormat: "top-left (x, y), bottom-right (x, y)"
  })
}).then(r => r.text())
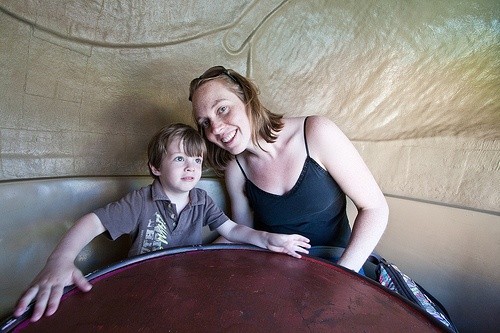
top-left (363, 253), bottom-right (461, 333)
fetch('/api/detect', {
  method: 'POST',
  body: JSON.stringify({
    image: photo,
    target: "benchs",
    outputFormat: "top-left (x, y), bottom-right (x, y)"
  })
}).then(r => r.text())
top-left (1, 175), bottom-right (228, 320)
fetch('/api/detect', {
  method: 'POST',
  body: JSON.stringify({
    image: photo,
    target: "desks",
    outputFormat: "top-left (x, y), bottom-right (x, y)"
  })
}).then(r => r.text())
top-left (0, 243), bottom-right (461, 333)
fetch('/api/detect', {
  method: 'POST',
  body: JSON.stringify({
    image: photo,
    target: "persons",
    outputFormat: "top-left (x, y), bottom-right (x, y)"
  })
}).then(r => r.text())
top-left (190, 65), bottom-right (390, 279)
top-left (12, 119), bottom-right (312, 324)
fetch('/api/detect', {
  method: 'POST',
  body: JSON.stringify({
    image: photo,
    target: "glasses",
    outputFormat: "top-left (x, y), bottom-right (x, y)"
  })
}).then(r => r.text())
top-left (188, 65), bottom-right (242, 100)
